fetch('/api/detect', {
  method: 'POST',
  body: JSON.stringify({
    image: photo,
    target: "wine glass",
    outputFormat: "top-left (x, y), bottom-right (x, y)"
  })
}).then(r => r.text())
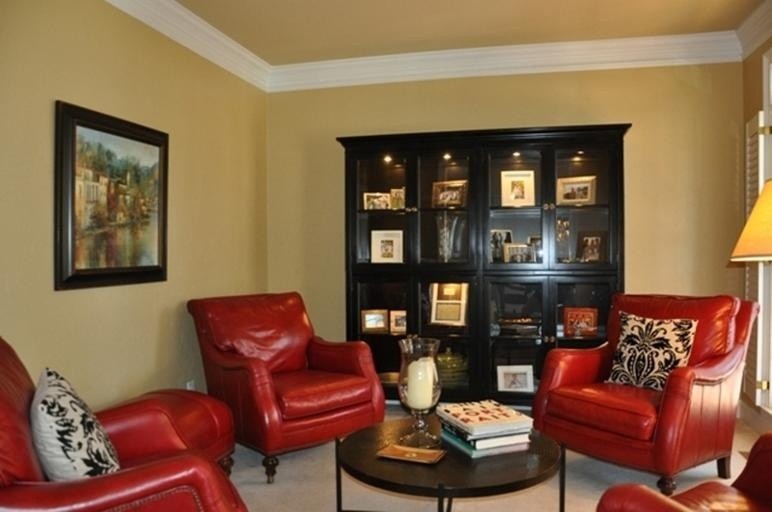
top-left (397, 338), bottom-right (442, 448)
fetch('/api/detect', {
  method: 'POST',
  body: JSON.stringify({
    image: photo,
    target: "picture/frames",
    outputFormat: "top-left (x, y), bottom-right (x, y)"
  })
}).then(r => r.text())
top-left (370, 228), bottom-right (405, 264)
top-left (431, 178), bottom-right (470, 209)
top-left (501, 242), bottom-right (538, 264)
top-left (489, 228), bottom-right (515, 264)
top-left (575, 229), bottom-right (609, 264)
top-left (360, 308), bottom-right (390, 336)
top-left (555, 173), bottom-right (598, 207)
top-left (388, 309), bottom-right (407, 336)
top-left (499, 169), bottom-right (537, 209)
top-left (525, 234), bottom-right (544, 264)
top-left (496, 364), bottom-right (536, 395)
top-left (361, 191), bottom-right (391, 211)
top-left (563, 305), bottom-right (600, 338)
top-left (426, 281), bottom-right (471, 329)
top-left (53, 99), bottom-right (170, 292)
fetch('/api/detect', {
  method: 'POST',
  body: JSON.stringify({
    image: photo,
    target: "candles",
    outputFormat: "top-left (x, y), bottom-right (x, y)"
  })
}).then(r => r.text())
top-left (406, 355), bottom-right (436, 410)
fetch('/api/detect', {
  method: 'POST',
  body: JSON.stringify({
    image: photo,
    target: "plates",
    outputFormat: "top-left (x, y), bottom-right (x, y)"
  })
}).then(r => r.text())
top-left (376, 444), bottom-right (446, 463)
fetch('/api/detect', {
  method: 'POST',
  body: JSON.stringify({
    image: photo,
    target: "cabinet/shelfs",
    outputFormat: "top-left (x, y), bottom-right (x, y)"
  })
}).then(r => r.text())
top-left (335, 122), bottom-right (634, 409)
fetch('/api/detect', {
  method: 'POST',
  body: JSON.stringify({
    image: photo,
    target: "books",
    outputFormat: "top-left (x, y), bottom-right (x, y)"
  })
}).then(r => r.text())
top-left (435, 399), bottom-right (534, 459)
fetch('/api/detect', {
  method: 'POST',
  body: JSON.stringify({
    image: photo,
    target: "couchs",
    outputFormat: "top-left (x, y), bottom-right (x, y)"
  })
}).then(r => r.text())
top-left (186, 290), bottom-right (387, 485)
top-left (529, 291), bottom-right (760, 499)
top-left (0, 335), bottom-right (250, 511)
top-left (592, 429), bottom-right (772, 512)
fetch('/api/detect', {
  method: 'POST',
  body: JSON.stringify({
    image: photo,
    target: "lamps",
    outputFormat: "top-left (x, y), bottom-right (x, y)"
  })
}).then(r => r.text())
top-left (729, 179), bottom-right (772, 263)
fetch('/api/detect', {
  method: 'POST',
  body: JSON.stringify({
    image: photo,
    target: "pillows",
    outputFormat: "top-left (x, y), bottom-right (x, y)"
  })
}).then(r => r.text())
top-left (604, 308), bottom-right (700, 391)
top-left (30, 364), bottom-right (121, 483)
